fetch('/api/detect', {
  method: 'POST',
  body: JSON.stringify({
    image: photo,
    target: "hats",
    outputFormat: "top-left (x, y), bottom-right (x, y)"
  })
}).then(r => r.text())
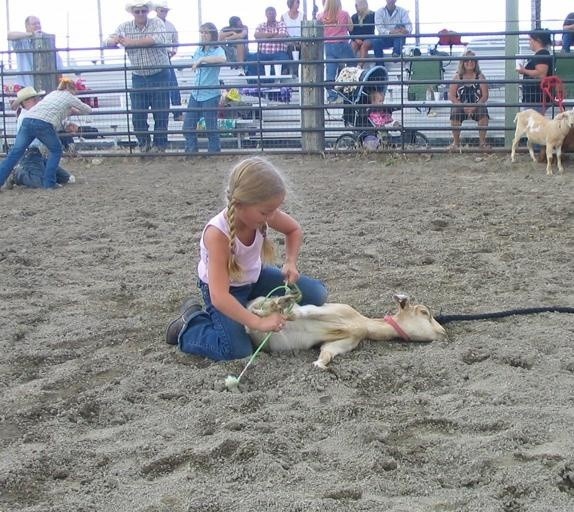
top-left (151, 1), bottom-right (170, 12)
top-left (11, 85), bottom-right (46, 110)
top-left (124, 0), bottom-right (152, 14)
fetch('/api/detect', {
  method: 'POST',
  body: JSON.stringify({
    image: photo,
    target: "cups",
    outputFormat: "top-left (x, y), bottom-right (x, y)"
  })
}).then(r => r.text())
top-left (274, 64), bottom-right (282, 75)
top-left (264, 65), bottom-right (272, 76)
top-left (434, 92), bottom-right (441, 102)
top-left (292, 50), bottom-right (300, 60)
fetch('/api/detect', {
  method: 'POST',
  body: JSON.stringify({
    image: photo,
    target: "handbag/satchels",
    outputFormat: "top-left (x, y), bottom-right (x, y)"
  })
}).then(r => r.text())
top-left (433, 29), bottom-right (463, 69)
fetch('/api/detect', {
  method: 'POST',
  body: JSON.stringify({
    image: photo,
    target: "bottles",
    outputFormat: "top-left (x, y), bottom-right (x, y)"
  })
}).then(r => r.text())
top-left (443, 85), bottom-right (449, 101)
top-left (421, 106), bottom-right (427, 118)
top-left (427, 44), bottom-right (432, 56)
top-left (425, 88), bottom-right (431, 102)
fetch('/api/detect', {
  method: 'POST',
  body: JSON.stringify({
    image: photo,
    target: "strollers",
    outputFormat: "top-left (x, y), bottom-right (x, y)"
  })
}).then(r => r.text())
top-left (324, 65), bottom-right (430, 151)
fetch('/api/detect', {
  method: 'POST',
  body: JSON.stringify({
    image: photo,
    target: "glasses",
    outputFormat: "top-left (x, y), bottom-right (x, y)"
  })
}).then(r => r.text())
top-left (132, 9), bottom-right (148, 14)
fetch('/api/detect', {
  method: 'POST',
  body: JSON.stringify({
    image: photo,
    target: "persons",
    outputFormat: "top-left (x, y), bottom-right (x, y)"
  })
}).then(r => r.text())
top-left (253, 7), bottom-right (290, 75)
top-left (514, 28), bottom-right (555, 115)
top-left (5, 15), bottom-right (64, 85)
top-left (164, 155), bottom-right (328, 363)
top-left (281, 0), bottom-right (305, 61)
top-left (102, 1), bottom-right (171, 150)
top-left (367, 91), bottom-right (402, 137)
top-left (151, 2), bottom-right (184, 122)
top-left (347, 1), bottom-right (374, 66)
top-left (0, 78), bottom-right (92, 191)
top-left (560, 12), bottom-right (574, 53)
top-left (218, 16), bottom-right (249, 77)
top-left (371, 1), bottom-right (413, 68)
top-left (3, 85), bottom-right (78, 190)
top-left (315, 1), bottom-right (356, 103)
top-left (445, 50), bottom-right (491, 150)
top-left (181, 22), bottom-right (226, 152)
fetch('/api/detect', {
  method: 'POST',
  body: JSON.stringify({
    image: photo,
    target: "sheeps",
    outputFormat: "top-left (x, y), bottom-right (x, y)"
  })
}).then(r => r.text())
top-left (243, 292), bottom-right (448, 371)
top-left (509, 106), bottom-right (574, 177)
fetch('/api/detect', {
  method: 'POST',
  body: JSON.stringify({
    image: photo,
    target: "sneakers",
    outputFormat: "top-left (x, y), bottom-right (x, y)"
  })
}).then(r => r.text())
top-left (165, 299), bottom-right (203, 347)
top-left (139, 146), bottom-right (164, 153)
top-left (174, 115), bottom-right (183, 121)
